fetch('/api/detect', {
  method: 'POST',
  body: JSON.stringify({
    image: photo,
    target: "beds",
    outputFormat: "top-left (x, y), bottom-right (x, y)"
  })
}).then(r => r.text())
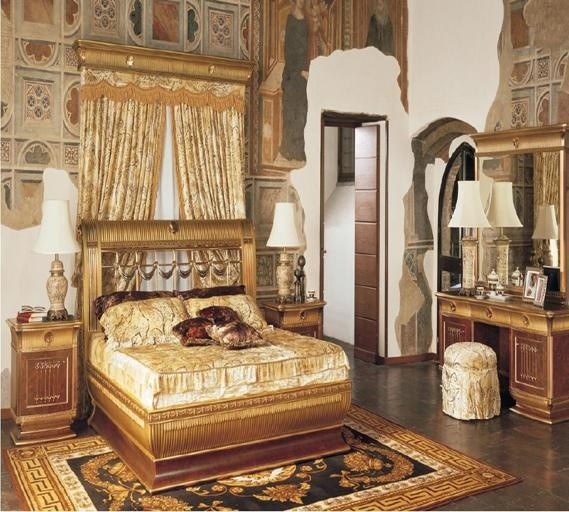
top-left (81, 219), bottom-right (352, 495)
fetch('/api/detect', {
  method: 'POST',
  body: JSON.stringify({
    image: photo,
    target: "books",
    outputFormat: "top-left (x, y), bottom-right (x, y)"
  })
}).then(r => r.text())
top-left (17, 317), bottom-right (42, 323)
top-left (18, 311), bottom-right (47, 317)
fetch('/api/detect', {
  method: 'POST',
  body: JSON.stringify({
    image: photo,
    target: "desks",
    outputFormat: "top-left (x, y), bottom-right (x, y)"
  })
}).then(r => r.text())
top-left (434, 283), bottom-right (568, 427)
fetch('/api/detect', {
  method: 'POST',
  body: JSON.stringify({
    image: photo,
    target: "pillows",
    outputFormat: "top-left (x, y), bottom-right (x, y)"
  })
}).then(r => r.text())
top-left (95, 290), bottom-right (277, 351)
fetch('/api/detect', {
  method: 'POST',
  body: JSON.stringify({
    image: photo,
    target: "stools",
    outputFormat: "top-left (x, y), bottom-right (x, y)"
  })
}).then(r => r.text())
top-left (440, 342), bottom-right (502, 422)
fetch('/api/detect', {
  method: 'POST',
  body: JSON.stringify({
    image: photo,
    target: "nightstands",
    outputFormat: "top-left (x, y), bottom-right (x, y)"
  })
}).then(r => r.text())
top-left (260, 297), bottom-right (330, 340)
top-left (5, 319), bottom-right (83, 443)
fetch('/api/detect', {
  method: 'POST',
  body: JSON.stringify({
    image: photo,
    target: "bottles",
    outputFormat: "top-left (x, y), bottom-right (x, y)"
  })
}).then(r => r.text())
top-left (487, 269), bottom-right (498, 290)
top-left (511, 266), bottom-right (524, 287)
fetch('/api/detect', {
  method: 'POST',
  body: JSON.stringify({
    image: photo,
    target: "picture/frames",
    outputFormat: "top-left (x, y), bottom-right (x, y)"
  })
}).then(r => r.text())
top-left (200, 2), bottom-right (239, 61)
top-left (533, 275), bottom-right (548, 308)
top-left (522, 266), bottom-right (545, 304)
top-left (81, 0), bottom-right (126, 45)
top-left (141, 1), bottom-right (189, 53)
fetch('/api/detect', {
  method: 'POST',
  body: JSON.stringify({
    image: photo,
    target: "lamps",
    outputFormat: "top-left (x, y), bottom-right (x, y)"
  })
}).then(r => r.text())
top-left (447, 179), bottom-right (491, 297)
top-left (33, 199), bottom-right (81, 319)
top-left (489, 179), bottom-right (524, 288)
top-left (265, 201), bottom-right (303, 301)
top-left (533, 204), bottom-right (560, 266)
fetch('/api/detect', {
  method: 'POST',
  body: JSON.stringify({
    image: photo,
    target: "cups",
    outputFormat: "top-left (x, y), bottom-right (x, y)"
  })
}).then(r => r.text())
top-left (490, 291), bottom-right (505, 296)
top-left (477, 286), bottom-right (484, 295)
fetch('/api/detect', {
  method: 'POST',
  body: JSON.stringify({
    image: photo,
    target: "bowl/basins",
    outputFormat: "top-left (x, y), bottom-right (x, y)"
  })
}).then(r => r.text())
top-left (488, 294), bottom-right (514, 304)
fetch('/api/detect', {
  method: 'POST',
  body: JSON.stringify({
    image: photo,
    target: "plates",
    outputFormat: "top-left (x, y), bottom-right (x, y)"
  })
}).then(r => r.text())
top-left (475, 295), bottom-right (487, 300)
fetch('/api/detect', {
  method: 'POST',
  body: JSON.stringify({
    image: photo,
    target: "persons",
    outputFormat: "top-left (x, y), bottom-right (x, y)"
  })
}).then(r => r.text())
top-left (365, 0), bottom-right (393, 56)
top-left (279, 0), bottom-right (309, 161)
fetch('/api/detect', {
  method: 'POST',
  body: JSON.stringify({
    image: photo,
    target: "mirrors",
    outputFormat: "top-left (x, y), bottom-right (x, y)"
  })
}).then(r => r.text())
top-left (469, 123), bottom-right (568, 304)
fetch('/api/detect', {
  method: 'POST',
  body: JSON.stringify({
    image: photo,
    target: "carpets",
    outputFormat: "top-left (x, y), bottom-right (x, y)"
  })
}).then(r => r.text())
top-left (5, 402), bottom-right (524, 510)
